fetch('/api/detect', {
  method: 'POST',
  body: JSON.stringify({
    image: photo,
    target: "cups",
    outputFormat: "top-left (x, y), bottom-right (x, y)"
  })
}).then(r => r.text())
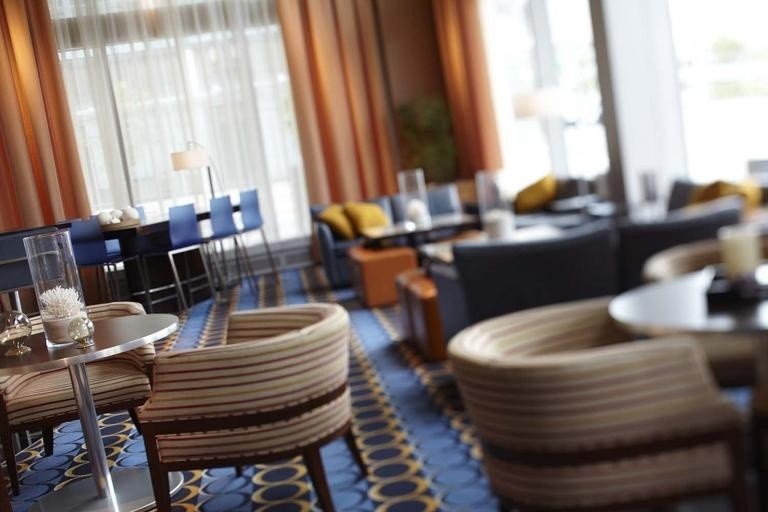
top-left (22, 229), bottom-right (91, 347)
top-left (395, 168), bottom-right (432, 231)
top-left (474, 167), bottom-right (520, 243)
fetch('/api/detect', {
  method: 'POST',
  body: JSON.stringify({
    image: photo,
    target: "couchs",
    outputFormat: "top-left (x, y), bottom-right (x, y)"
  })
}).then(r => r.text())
top-left (307, 180), bottom-right (766, 360)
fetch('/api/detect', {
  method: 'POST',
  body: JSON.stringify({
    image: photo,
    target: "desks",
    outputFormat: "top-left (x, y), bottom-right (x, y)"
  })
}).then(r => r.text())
top-left (605, 261), bottom-right (766, 497)
top-left (2, 314), bottom-right (184, 512)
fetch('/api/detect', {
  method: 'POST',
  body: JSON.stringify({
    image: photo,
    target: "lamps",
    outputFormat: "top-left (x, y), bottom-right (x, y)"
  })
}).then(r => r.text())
top-left (169, 139), bottom-right (215, 199)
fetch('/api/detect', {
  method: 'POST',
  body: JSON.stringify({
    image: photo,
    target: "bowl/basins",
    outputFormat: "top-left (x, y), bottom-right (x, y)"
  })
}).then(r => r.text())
top-left (99, 219), bottom-right (140, 232)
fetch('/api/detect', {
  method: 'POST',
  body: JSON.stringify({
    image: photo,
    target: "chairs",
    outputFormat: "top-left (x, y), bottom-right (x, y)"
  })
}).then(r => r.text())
top-left (1, 302), bottom-right (157, 497)
top-left (447, 298), bottom-right (747, 512)
top-left (0, 179), bottom-right (280, 311)
top-left (137, 301), bottom-right (369, 512)
